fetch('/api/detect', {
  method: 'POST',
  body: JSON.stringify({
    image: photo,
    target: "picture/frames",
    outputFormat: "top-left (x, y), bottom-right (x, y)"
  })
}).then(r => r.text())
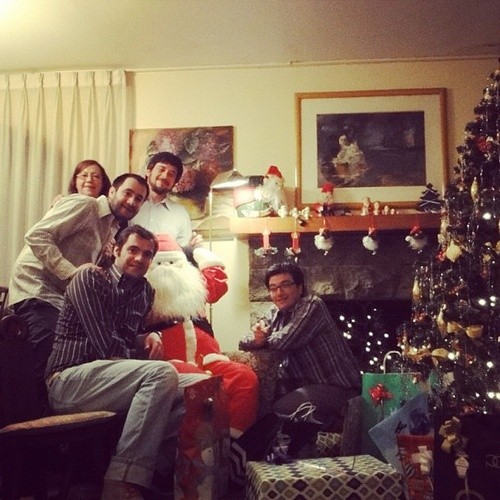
top-left (294, 88), bottom-right (449, 209)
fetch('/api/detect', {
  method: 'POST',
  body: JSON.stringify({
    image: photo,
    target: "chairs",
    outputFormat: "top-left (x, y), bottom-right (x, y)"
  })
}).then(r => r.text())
top-left (0, 316), bottom-right (118, 500)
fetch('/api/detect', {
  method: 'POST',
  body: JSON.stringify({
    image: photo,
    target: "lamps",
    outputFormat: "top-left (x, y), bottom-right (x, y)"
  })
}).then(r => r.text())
top-left (209, 170), bottom-right (248, 326)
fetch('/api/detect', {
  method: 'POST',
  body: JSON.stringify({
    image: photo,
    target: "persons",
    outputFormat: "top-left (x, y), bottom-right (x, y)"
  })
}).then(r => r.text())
top-left (123, 152), bottom-right (193, 277)
top-left (39, 224), bottom-right (213, 500)
top-left (49, 160), bottom-right (112, 213)
top-left (8, 173), bottom-right (150, 363)
top-left (242, 261), bottom-right (364, 459)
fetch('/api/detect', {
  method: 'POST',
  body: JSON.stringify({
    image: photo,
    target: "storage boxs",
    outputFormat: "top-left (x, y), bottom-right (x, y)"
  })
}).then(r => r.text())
top-left (243, 455), bottom-right (403, 500)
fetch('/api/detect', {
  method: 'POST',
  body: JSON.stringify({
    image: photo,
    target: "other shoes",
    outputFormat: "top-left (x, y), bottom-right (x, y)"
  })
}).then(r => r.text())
top-left (101, 479), bottom-right (146, 500)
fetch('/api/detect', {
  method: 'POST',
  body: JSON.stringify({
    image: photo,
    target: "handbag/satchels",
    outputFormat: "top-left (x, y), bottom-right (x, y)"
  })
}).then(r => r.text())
top-left (226, 402), bottom-right (322, 486)
top-left (360, 350), bottom-right (421, 461)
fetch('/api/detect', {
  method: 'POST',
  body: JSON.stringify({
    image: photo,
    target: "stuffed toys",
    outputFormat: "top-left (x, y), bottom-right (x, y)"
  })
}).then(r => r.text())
top-left (137, 233), bottom-right (260, 499)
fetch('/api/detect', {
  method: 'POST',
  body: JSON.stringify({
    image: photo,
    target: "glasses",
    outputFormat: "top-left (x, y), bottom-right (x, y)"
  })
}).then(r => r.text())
top-left (269, 280), bottom-right (297, 293)
top-left (76, 173), bottom-right (103, 181)
